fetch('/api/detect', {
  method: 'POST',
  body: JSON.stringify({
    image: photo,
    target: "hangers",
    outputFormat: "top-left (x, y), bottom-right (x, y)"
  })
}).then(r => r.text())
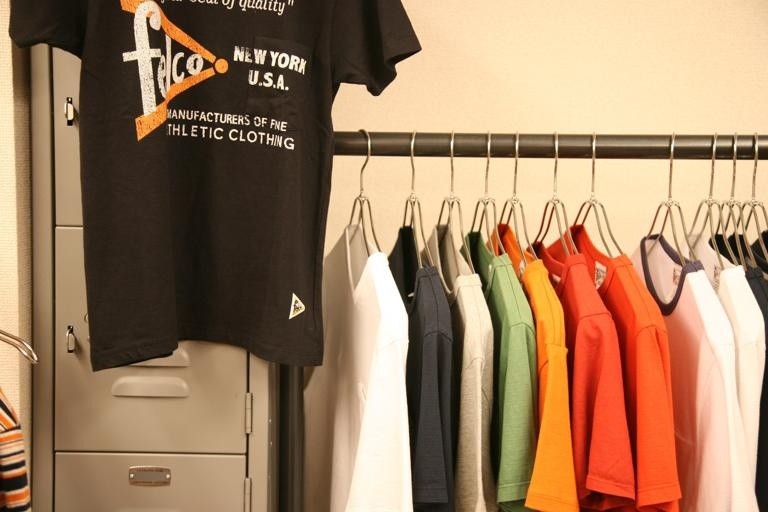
top-left (348, 128), bottom-right (768, 270)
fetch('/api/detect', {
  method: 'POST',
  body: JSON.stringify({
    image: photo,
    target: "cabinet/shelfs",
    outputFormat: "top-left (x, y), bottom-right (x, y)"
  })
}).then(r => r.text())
top-left (30, 42), bottom-right (280, 512)
